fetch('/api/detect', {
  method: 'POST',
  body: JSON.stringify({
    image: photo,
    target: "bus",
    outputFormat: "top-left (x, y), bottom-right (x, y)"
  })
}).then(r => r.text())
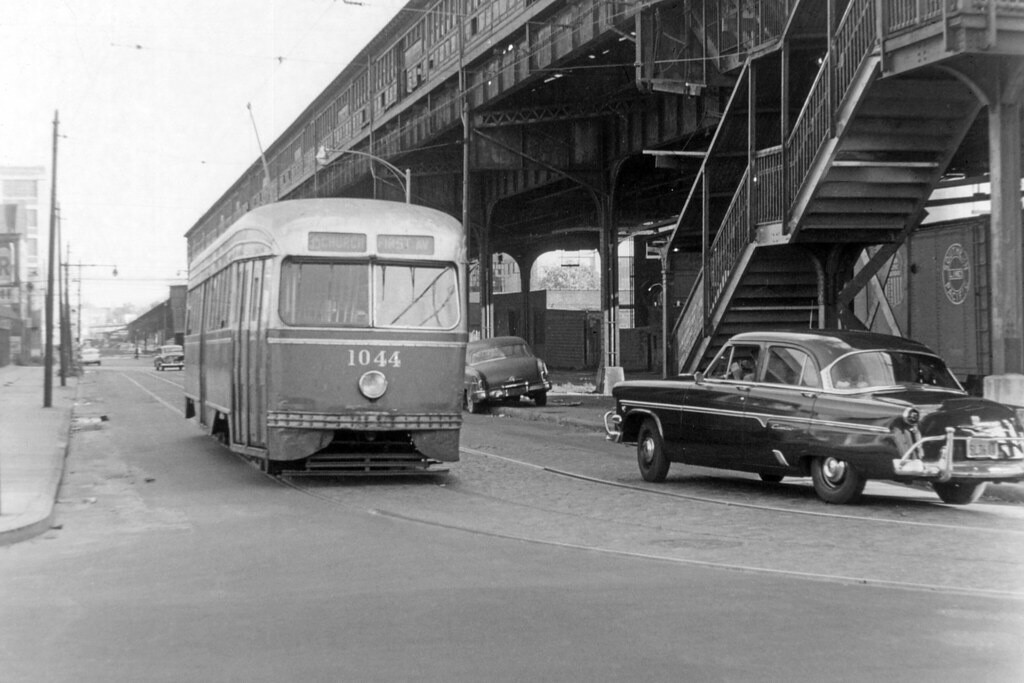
top-left (182, 198), bottom-right (468, 477)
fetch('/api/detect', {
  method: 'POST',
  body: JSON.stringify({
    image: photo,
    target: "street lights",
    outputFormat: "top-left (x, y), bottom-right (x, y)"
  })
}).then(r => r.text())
top-left (57, 264), bottom-right (118, 341)
top-left (316, 144), bottom-right (413, 202)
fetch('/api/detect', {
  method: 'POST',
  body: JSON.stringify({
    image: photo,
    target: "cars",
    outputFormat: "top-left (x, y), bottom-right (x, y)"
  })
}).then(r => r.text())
top-left (463, 337), bottom-right (553, 413)
top-left (153, 345), bottom-right (185, 371)
top-left (83, 348), bottom-right (101, 365)
top-left (604, 329), bottom-right (1022, 505)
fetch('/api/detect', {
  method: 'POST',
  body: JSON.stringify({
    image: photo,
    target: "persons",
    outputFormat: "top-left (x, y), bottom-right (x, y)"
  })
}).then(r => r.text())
top-left (731, 348), bottom-right (759, 382)
